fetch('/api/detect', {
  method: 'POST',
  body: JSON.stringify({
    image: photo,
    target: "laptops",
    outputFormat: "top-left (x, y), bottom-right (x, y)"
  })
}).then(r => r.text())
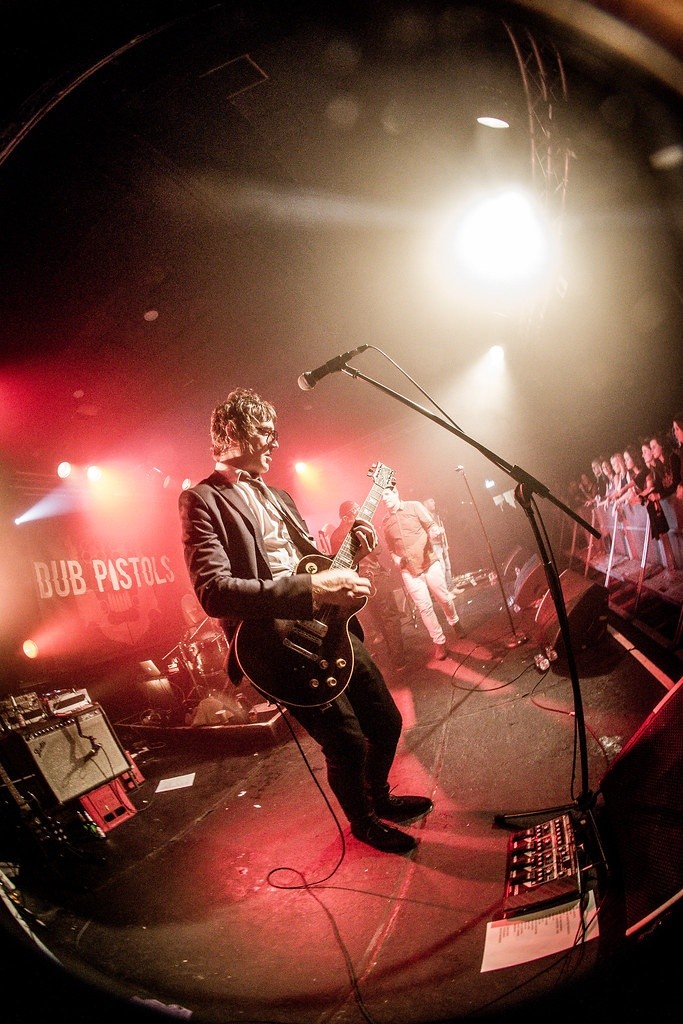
top-left (139, 660), bottom-right (169, 676)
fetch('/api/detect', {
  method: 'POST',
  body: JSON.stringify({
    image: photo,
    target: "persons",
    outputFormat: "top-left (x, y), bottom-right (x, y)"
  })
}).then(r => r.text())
top-left (180, 581), bottom-right (210, 640)
top-left (378, 481), bottom-right (468, 662)
top-left (420, 493), bottom-right (467, 600)
top-left (330, 499), bottom-right (417, 675)
top-left (549, 415), bottom-right (683, 582)
top-left (176, 384), bottom-right (435, 859)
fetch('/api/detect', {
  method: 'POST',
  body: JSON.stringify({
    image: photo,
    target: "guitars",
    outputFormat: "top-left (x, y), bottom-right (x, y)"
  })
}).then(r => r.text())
top-left (234, 458), bottom-right (399, 714)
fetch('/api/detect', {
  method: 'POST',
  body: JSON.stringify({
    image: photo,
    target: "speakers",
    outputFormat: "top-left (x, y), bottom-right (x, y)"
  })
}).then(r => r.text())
top-left (534, 568), bottom-right (609, 652)
top-left (599, 675), bottom-right (683, 942)
top-left (500, 545), bottom-right (529, 578)
top-left (514, 553), bottom-right (550, 608)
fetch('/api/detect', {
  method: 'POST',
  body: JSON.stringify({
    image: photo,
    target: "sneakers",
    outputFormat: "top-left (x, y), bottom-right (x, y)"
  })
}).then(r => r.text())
top-left (350, 815), bottom-right (416, 852)
top-left (396, 656), bottom-right (412, 670)
top-left (373, 792), bottom-right (433, 816)
top-left (451, 588), bottom-right (465, 595)
top-left (453, 622), bottom-right (466, 638)
top-left (449, 592), bottom-right (457, 600)
top-left (434, 644), bottom-right (447, 660)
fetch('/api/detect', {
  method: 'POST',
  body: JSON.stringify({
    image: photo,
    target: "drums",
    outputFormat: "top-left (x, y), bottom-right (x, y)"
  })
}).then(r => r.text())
top-left (187, 636), bottom-right (227, 680)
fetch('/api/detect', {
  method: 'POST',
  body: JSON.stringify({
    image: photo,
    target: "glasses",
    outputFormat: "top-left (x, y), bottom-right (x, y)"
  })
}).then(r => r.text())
top-left (255, 426), bottom-right (279, 445)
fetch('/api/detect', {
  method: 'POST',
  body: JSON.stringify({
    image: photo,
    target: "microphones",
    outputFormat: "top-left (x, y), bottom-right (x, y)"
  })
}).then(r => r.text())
top-left (298, 344), bottom-right (368, 392)
